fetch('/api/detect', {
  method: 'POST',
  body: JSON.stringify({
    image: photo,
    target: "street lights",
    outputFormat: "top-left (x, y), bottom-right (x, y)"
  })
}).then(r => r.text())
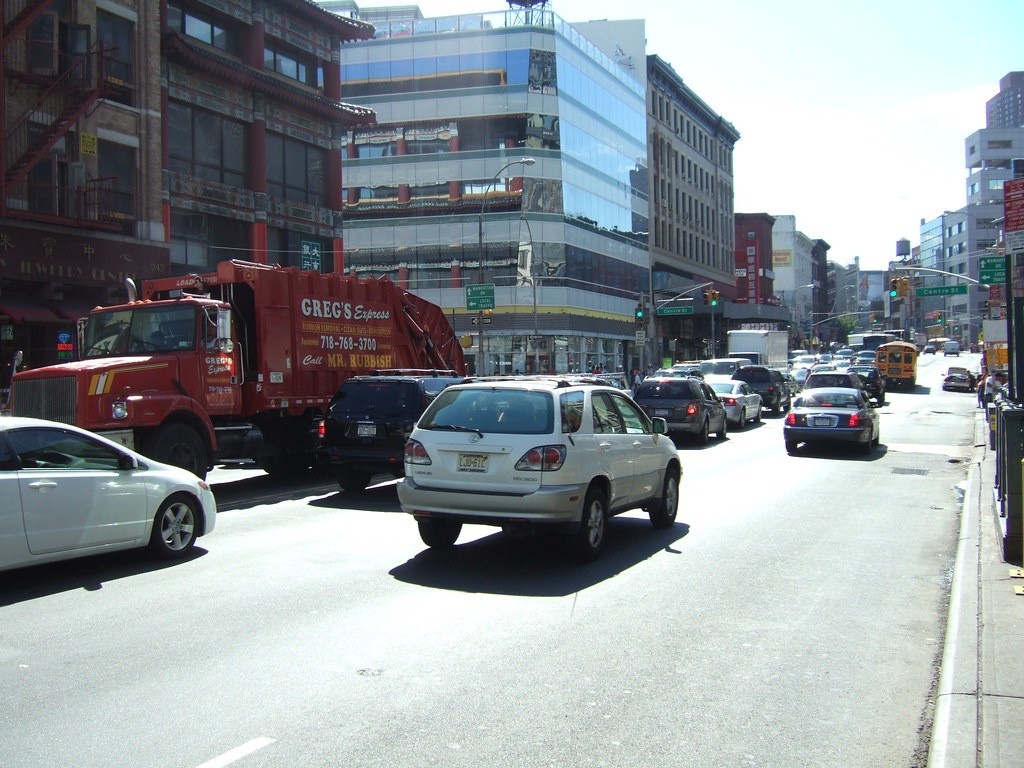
top-left (477, 157), bottom-right (540, 376)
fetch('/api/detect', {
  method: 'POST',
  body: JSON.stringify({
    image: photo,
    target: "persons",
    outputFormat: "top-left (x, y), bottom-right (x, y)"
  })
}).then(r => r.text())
top-left (200, 319), bottom-right (219, 350)
top-left (539, 363), bottom-right (556, 375)
top-left (494, 371), bottom-right (499, 376)
top-left (150, 331), bottom-right (178, 350)
top-left (631, 362), bottom-right (655, 396)
top-left (591, 362), bottom-right (608, 374)
top-left (513, 369), bottom-right (522, 376)
top-left (976, 372), bottom-right (1009, 423)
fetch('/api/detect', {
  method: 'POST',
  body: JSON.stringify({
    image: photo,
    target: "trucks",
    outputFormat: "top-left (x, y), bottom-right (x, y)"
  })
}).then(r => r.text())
top-left (726, 328), bottom-right (789, 367)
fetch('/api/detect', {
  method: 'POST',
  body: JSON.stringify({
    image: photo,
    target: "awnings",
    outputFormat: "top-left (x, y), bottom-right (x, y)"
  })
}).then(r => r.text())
top-left (0, 298), bottom-right (73, 326)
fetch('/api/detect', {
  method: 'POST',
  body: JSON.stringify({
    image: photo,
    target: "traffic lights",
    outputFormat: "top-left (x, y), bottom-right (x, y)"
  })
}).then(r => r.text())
top-left (634, 307), bottom-right (644, 330)
top-left (703, 289), bottom-right (721, 306)
top-left (937, 314), bottom-right (941, 323)
top-left (890, 278), bottom-right (897, 297)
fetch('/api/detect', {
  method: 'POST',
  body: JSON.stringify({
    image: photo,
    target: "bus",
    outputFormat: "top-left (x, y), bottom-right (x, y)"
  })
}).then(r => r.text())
top-left (877, 340), bottom-right (919, 389)
top-left (863, 333), bottom-right (895, 350)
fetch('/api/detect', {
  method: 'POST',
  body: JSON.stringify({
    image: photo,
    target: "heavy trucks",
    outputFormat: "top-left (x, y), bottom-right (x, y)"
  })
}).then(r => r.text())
top-left (8, 257), bottom-right (467, 481)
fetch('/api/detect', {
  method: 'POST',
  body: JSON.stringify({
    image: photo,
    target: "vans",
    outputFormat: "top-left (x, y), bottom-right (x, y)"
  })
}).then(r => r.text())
top-left (730, 364), bottom-right (792, 416)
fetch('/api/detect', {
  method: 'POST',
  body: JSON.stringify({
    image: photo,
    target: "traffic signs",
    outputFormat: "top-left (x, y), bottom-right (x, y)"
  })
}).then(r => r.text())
top-left (979, 256), bottom-right (1006, 284)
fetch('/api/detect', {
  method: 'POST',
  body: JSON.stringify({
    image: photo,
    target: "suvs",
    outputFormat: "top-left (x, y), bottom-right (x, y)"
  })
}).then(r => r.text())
top-left (398, 374), bottom-right (684, 557)
top-left (632, 374), bottom-right (728, 444)
top-left (313, 365), bottom-right (469, 491)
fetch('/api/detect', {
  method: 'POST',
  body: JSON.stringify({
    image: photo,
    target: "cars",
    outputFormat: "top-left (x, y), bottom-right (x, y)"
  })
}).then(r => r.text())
top-left (703, 378), bottom-right (763, 428)
top-left (781, 386), bottom-right (880, 455)
top-left (788, 348), bottom-right (877, 389)
top-left (0, 415), bottom-right (217, 572)
top-left (654, 358), bottom-right (753, 382)
top-left (923, 341), bottom-right (980, 392)
